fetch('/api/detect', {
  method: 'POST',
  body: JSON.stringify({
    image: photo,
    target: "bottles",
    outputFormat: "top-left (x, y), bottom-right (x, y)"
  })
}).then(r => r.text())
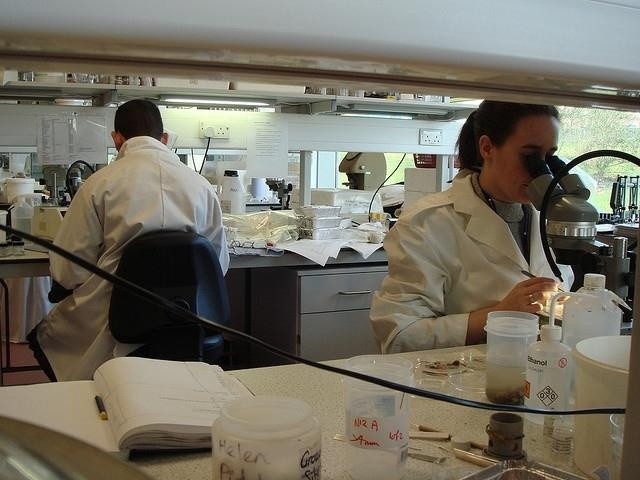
top-left (563, 273), bottom-right (620, 404)
top-left (526, 325), bottom-right (572, 424)
top-left (342, 354), bottom-right (409, 479)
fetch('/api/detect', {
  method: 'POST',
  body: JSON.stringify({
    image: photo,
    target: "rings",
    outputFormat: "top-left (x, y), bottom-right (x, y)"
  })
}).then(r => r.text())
top-left (529, 293), bottom-right (536, 304)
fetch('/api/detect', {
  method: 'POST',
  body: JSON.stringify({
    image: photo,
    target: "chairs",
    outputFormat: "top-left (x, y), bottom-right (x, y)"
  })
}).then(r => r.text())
top-left (109, 231), bottom-right (231, 361)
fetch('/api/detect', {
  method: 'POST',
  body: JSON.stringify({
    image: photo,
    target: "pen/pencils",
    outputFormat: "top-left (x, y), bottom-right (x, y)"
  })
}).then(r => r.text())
top-left (94, 396), bottom-right (108, 420)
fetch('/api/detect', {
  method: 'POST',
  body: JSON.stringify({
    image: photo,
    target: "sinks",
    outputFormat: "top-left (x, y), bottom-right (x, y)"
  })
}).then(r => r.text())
top-left (463, 461), bottom-right (586, 480)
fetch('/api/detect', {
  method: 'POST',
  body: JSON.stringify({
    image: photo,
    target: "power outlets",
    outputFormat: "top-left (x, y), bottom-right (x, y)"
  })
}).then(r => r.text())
top-left (419, 129), bottom-right (442, 145)
top-left (200, 121), bottom-right (231, 138)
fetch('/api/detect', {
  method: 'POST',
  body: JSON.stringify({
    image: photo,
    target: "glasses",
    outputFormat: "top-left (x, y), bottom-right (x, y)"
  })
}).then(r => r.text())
top-left (231, 241), bottom-right (255, 248)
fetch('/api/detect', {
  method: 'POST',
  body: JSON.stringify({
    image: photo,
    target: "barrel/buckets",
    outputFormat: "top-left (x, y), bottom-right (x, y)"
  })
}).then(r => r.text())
top-left (216, 170), bottom-right (246, 214)
top-left (571, 333), bottom-right (632, 479)
top-left (252, 177), bottom-right (274, 200)
top-left (7, 178), bottom-right (35, 203)
top-left (11, 197), bottom-right (34, 244)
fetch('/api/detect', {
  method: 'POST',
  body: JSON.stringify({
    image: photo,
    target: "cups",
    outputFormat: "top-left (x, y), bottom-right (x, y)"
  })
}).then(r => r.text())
top-left (212, 397), bottom-right (323, 480)
top-left (484, 311), bottom-right (541, 406)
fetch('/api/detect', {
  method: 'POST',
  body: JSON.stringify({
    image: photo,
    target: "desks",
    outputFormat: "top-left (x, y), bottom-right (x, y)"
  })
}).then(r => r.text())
top-left (0, 246), bottom-right (389, 387)
top-left (129, 344), bottom-right (589, 479)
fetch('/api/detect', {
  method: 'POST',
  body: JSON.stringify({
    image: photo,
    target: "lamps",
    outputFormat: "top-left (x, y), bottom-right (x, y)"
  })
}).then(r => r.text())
top-left (160, 93), bottom-right (277, 107)
top-left (341, 110), bottom-right (415, 119)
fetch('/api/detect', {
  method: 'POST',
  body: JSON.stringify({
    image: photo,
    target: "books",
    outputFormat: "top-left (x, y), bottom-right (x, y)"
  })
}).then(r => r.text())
top-left (0, 355), bottom-right (256, 458)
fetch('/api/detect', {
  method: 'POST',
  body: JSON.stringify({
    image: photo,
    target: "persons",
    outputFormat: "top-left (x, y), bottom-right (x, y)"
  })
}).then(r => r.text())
top-left (30, 99), bottom-right (232, 382)
top-left (368, 99), bottom-right (571, 355)
